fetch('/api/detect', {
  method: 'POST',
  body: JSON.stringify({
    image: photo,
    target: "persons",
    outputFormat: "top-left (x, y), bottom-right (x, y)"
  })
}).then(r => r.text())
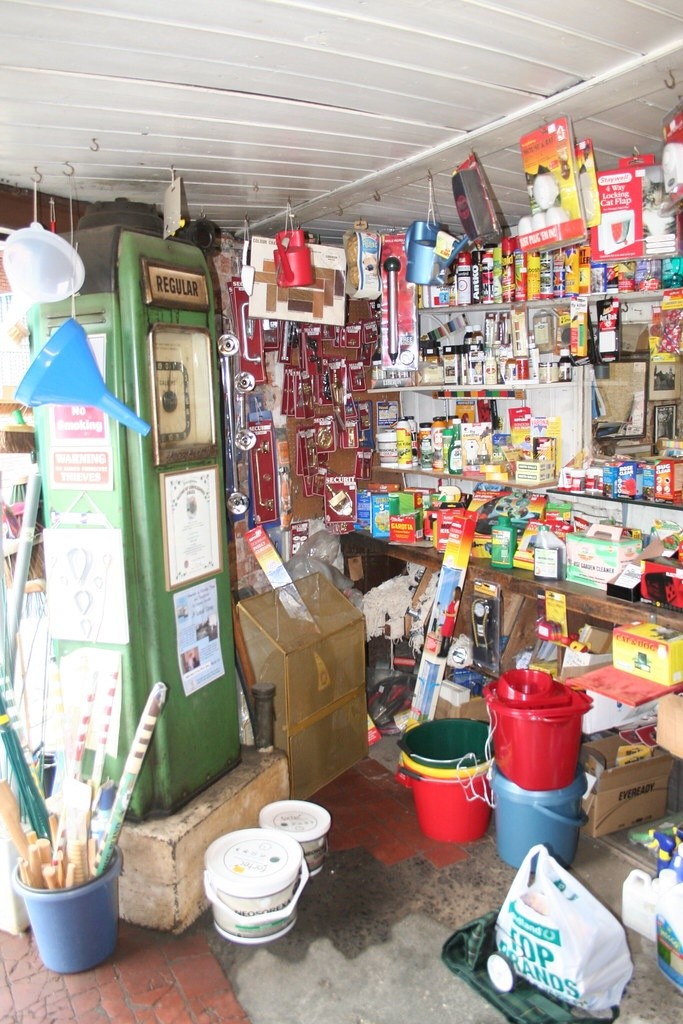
top-left (431, 601), bottom-right (440, 632)
top-left (655, 369), bottom-right (669, 390)
top-left (188, 652), bottom-right (195, 671)
top-left (658, 407), bottom-right (673, 439)
top-left (436, 586), bottom-right (461, 658)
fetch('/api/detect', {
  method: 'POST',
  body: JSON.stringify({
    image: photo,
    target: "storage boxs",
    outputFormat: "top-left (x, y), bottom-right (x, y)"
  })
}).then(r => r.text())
top-left (580, 733), bottom-right (672, 838)
top-left (612, 620), bottom-right (683, 686)
top-left (559, 646), bottom-right (613, 686)
top-left (435, 669), bottom-right (494, 720)
top-left (354, 345), bottom-right (683, 609)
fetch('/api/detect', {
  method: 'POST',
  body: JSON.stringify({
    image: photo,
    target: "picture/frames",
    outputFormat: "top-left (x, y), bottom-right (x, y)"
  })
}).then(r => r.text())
top-left (648, 353), bottom-right (681, 400)
top-left (654, 405), bottom-right (678, 454)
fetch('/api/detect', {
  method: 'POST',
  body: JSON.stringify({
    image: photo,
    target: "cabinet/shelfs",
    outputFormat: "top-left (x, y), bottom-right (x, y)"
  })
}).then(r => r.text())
top-left (352, 286), bottom-right (682, 644)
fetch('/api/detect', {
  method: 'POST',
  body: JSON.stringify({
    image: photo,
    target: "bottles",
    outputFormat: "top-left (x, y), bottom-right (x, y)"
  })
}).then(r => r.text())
top-left (396, 415), bottom-right (470, 475)
top-left (489, 516), bottom-right (517, 569)
top-left (533, 526), bottom-right (566, 581)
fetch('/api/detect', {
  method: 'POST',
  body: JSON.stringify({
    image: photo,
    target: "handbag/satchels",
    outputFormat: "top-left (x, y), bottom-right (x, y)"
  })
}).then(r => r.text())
top-left (495, 845), bottom-right (635, 1011)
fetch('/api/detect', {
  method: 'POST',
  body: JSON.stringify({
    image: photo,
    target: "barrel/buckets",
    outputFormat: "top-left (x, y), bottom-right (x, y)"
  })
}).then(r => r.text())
top-left (12, 843), bottom-right (124, 974)
top-left (203, 828), bottom-right (309, 945)
top-left (620, 870), bottom-right (678, 942)
top-left (397, 718), bottom-right (493, 843)
top-left (654, 881), bottom-right (683, 995)
top-left (482, 669), bottom-right (591, 791)
top-left (259, 800), bottom-right (332, 878)
top-left (492, 760), bottom-right (588, 870)
